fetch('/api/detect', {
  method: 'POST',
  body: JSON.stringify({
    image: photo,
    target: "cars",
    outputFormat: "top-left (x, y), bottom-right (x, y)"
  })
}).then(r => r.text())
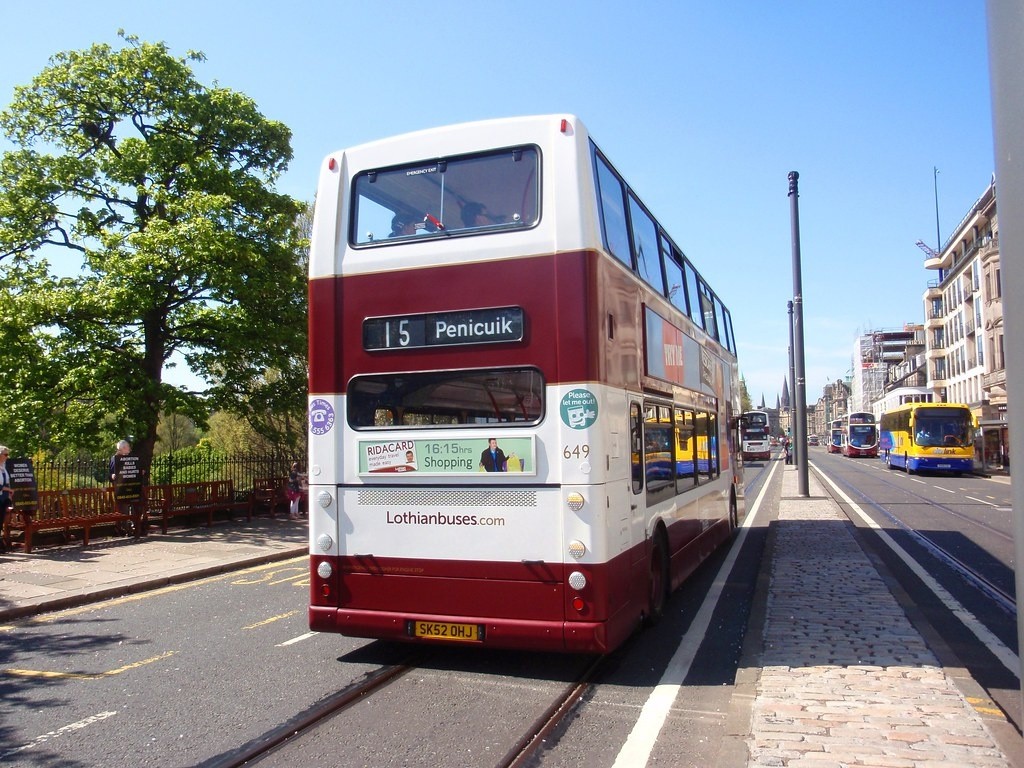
top-left (770, 435), bottom-right (786, 447)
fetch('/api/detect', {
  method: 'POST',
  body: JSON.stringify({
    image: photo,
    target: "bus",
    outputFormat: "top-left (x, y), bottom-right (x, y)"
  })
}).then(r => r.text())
top-left (305, 110), bottom-right (740, 661)
top-left (741, 410), bottom-right (772, 461)
top-left (878, 401), bottom-right (979, 477)
top-left (841, 412), bottom-right (880, 458)
top-left (825, 420), bottom-right (856, 454)
top-left (807, 434), bottom-right (819, 446)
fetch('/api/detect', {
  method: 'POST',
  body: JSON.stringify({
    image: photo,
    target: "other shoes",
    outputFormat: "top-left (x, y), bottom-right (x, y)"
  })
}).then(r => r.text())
top-left (294, 514), bottom-right (302, 518)
top-left (289, 514), bottom-right (297, 519)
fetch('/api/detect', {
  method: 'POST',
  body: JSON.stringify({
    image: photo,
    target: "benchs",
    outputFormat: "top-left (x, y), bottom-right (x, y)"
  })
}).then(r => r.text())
top-left (3, 477), bottom-right (290, 554)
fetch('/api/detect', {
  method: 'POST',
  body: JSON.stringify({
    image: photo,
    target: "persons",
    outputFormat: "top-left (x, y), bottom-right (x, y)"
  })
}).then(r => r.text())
top-left (389, 214), bottom-right (415, 237)
top-left (754, 415), bottom-right (761, 422)
top-left (405, 451), bottom-right (414, 463)
top-left (916, 427), bottom-right (932, 441)
top-left (0, 447), bottom-right (14, 537)
top-left (782, 437), bottom-right (792, 464)
top-left (461, 202), bottom-right (490, 227)
top-left (479, 439), bottom-right (513, 472)
top-left (289, 462), bottom-right (302, 520)
top-left (108, 440), bottom-right (144, 535)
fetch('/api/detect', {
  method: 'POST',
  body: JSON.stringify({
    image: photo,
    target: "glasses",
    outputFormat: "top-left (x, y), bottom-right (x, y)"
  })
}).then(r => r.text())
top-left (478, 213), bottom-right (490, 219)
top-left (2, 454), bottom-right (8, 457)
